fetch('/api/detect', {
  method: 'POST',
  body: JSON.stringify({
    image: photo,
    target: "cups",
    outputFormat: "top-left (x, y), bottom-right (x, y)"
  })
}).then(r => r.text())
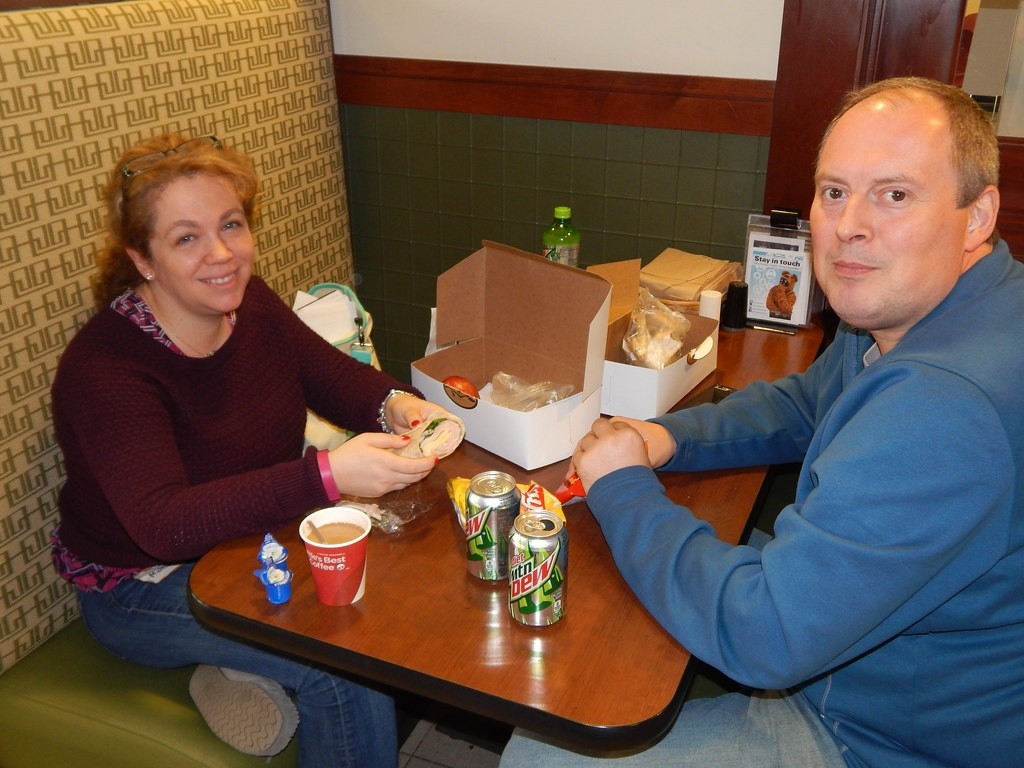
top-left (299, 507), bottom-right (372, 607)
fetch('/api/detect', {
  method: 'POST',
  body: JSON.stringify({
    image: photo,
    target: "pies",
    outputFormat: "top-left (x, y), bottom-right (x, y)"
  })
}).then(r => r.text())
top-left (384, 413), bottom-right (466, 462)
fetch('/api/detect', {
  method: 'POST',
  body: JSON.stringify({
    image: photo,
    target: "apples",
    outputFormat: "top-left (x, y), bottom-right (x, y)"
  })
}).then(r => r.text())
top-left (442, 376), bottom-right (480, 400)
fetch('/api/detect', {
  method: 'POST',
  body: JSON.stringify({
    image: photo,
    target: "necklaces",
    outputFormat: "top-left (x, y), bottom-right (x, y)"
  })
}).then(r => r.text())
top-left (142, 284), bottom-right (210, 358)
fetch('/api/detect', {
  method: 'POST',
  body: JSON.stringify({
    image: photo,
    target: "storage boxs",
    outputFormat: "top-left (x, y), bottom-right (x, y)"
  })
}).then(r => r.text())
top-left (409, 241), bottom-right (613, 469)
top-left (574, 259), bottom-right (720, 424)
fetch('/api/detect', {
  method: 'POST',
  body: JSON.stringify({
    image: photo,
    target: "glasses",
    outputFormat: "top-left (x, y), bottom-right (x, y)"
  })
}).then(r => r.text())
top-left (121, 136), bottom-right (224, 218)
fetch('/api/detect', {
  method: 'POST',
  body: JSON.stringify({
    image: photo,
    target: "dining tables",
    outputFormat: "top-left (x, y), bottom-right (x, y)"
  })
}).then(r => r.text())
top-left (187, 299), bottom-right (822, 758)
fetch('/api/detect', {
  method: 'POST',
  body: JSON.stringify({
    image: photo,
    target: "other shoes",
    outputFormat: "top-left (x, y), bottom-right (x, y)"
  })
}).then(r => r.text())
top-left (188, 665), bottom-right (300, 756)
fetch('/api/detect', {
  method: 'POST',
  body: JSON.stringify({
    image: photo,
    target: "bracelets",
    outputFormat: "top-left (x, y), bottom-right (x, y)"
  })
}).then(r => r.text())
top-left (316, 445), bottom-right (341, 503)
top-left (376, 389), bottom-right (413, 434)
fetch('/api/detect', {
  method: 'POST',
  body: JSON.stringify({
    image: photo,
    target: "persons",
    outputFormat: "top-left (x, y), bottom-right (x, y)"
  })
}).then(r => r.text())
top-left (49, 131), bottom-right (450, 767)
top-left (496, 77), bottom-right (1024, 768)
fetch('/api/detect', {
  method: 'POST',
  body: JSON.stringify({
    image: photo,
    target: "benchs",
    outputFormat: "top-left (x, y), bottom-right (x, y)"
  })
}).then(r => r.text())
top-left (0, 1), bottom-right (359, 768)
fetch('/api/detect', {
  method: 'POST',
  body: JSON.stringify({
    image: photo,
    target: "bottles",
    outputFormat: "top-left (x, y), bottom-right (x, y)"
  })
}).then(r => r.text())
top-left (543, 206), bottom-right (579, 269)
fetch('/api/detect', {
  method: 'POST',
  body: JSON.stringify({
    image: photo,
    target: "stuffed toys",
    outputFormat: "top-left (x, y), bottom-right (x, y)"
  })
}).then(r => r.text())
top-left (766, 271), bottom-right (798, 319)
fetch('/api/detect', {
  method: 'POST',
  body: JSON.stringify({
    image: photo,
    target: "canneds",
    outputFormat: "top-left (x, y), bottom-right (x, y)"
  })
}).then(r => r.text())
top-left (507, 509), bottom-right (571, 626)
top-left (464, 470), bottom-right (521, 581)
